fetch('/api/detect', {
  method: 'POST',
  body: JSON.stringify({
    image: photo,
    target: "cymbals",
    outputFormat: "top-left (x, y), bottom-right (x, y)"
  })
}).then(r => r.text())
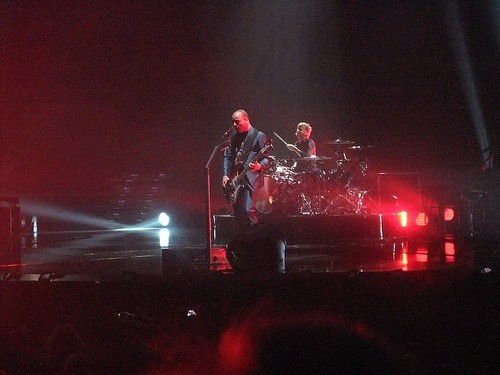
top-left (350, 145), bottom-right (373, 150)
top-left (296, 155), bottom-right (331, 161)
top-left (325, 140), bottom-right (355, 145)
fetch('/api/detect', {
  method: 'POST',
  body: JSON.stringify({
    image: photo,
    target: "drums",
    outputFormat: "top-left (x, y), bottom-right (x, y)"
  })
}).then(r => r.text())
top-left (296, 168), bottom-right (323, 196)
top-left (254, 169), bottom-right (301, 216)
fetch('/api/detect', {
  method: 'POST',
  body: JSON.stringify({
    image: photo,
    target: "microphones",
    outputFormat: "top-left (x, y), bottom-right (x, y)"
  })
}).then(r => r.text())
top-left (224, 125), bottom-right (235, 136)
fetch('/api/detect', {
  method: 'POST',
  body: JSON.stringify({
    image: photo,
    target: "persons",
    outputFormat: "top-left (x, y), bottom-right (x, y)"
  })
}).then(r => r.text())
top-left (221, 109), bottom-right (269, 229)
top-left (286, 122), bottom-right (317, 161)
top-left (0, 308), bottom-right (500, 375)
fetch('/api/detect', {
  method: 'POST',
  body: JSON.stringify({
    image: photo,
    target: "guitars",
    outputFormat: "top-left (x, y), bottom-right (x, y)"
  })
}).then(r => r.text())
top-left (223, 139), bottom-right (273, 206)
